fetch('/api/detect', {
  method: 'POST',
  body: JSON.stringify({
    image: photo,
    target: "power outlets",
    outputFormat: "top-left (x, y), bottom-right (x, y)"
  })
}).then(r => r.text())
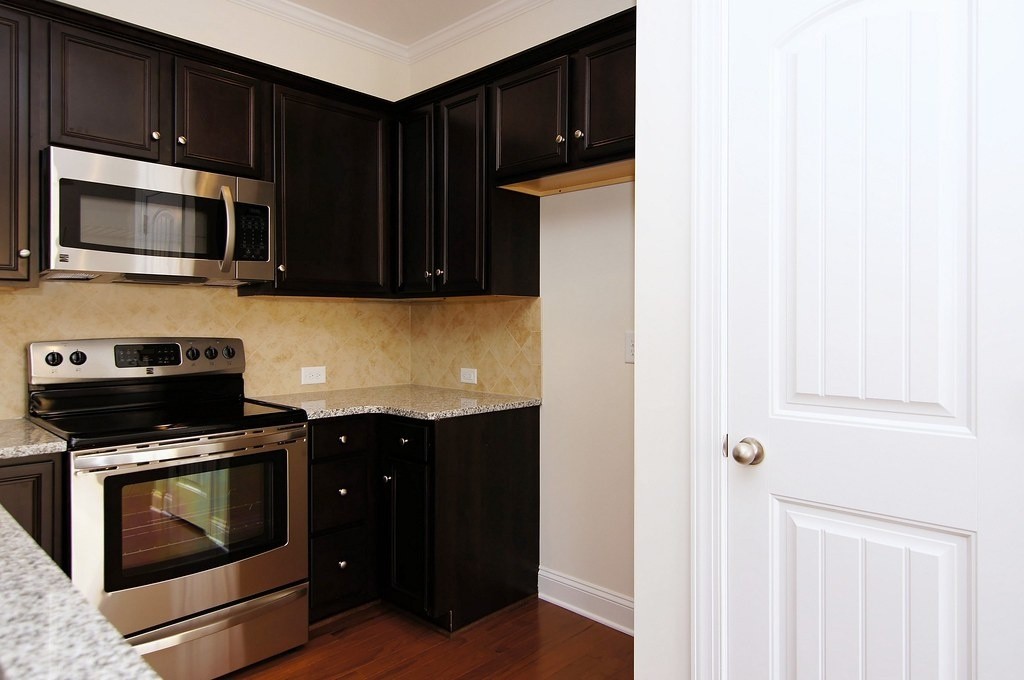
top-left (625, 331), bottom-right (634, 364)
top-left (460, 368), bottom-right (477, 385)
top-left (301, 366), bottom-right (326, 385)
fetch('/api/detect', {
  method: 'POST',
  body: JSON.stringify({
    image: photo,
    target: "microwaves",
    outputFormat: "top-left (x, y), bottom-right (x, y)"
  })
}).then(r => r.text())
top-left (39, 145), bottom-right (275, 280)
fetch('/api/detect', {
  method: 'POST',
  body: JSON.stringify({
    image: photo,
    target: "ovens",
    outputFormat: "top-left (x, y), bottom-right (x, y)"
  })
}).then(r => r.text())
top-left (68, 421), bottom-right (308, 680)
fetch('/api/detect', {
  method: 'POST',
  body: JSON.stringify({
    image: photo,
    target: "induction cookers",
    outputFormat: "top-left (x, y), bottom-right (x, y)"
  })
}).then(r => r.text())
top-left (25, 396), bottom-right (307, 449)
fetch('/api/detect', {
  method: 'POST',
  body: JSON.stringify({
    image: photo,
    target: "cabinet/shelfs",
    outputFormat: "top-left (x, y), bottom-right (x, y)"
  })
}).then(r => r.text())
top-left (486, 5), bottom-right (635, 197)
top-left (308, 413), bottom-right (380, 625)
top-left (237, 68), bottom-right (393, 301)
top-left (0, 0), bottom-right (40, 288)
top-left (375, 407), bottom-right (540, 633)
top-left (29, 0), bottom-right (275, 182)
top-left (394, 66), bottom-right (540, 303)
